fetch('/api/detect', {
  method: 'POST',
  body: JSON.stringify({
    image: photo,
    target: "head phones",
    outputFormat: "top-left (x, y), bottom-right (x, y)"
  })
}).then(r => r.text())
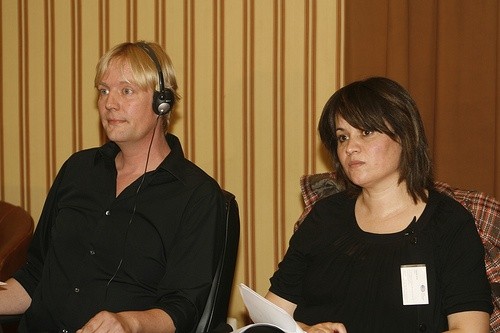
top-left (136, 42), bottom-right (174, 115)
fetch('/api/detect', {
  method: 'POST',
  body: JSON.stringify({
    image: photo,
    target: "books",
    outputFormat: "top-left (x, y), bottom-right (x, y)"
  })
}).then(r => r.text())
top-left (235, 283), bottom-right (306, 333)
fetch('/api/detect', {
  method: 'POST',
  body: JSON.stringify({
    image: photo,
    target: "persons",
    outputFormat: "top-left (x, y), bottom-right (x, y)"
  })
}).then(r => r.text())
top-left (264, 77), bottom-right (494, 333)
top-left (0, 42), bottom-right (226, 333)
